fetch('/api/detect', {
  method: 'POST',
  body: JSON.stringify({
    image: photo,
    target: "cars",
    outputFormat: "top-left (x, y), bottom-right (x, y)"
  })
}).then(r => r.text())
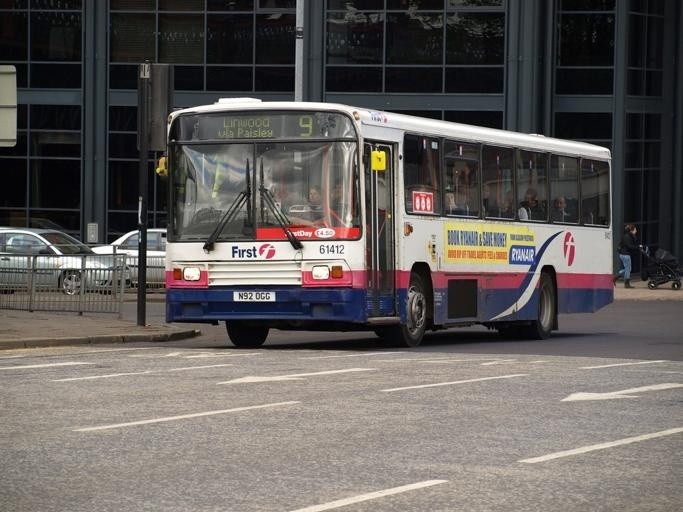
top-left (90, 228), bottom-right (167, 288)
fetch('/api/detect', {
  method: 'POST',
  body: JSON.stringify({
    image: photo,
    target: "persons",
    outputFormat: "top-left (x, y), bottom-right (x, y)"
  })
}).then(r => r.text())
top-left (614, 223), bottom-right (642, 288)
top-left (446, 183), bottom-right (569, 222)
top-left (306, 169), bottom-right (386, 288)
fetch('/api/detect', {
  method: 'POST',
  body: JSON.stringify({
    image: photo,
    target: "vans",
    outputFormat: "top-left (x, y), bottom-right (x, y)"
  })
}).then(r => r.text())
top-left (0, 228), bottom-right (133, 295)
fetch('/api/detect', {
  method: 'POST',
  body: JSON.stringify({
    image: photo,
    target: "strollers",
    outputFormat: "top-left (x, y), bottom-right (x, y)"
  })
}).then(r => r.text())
top-left (640, 245), bottom-right (681, 290)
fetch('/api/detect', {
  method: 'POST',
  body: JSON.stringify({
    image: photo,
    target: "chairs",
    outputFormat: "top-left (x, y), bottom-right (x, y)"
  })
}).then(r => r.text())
top-left (445, 204), bottom-right (593, 225)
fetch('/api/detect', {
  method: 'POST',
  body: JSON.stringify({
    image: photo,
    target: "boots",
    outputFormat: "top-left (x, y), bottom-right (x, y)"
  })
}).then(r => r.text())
top-left (623, 279), bottom-right (634, 288)
top-left (613, 272), bottom-right (620, 285)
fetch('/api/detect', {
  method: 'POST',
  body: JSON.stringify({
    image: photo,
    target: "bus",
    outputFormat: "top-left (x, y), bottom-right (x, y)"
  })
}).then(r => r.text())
top-left (166, 96), bottom-right (614, 348)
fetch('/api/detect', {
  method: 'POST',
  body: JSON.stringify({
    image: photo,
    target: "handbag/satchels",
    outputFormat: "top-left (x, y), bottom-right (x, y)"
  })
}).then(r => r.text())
top-left (617, 241), bottom-right (623, 253)
